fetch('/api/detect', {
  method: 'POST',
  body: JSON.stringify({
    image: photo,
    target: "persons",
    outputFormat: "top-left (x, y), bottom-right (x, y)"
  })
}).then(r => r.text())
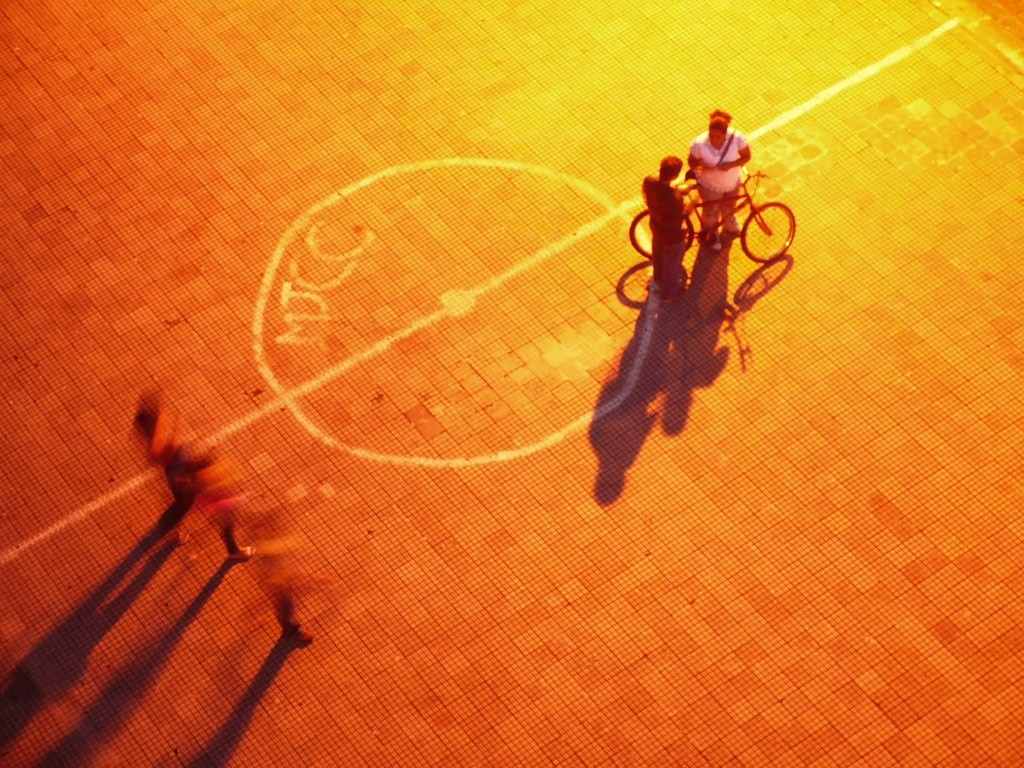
top-left (133, 396), bottom-right (310, 648)
top-left (690, 111), bottom-right (751, 251)
top-left (643, 156), bottom-right (688, 293)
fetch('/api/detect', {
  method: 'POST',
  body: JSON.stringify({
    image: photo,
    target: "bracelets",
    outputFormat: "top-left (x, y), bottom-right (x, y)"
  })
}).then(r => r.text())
top-left (737, 159), bottom-right (741, 166)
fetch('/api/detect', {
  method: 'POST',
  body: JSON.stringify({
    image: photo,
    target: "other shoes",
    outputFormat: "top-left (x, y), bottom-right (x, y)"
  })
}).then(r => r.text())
top-left (710, 237), bottom-right (722, 251)
top-left (722, 221), bottom-right (743, 235)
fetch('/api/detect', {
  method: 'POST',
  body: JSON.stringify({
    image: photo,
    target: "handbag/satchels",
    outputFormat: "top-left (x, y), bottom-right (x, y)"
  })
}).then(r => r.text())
top-left (685, 169), bottom-right (697, 181)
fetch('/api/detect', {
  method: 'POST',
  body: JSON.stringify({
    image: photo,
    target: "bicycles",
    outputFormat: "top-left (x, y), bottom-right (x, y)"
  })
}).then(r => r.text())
top-left (629, 170), bottom-right (795, 264)
